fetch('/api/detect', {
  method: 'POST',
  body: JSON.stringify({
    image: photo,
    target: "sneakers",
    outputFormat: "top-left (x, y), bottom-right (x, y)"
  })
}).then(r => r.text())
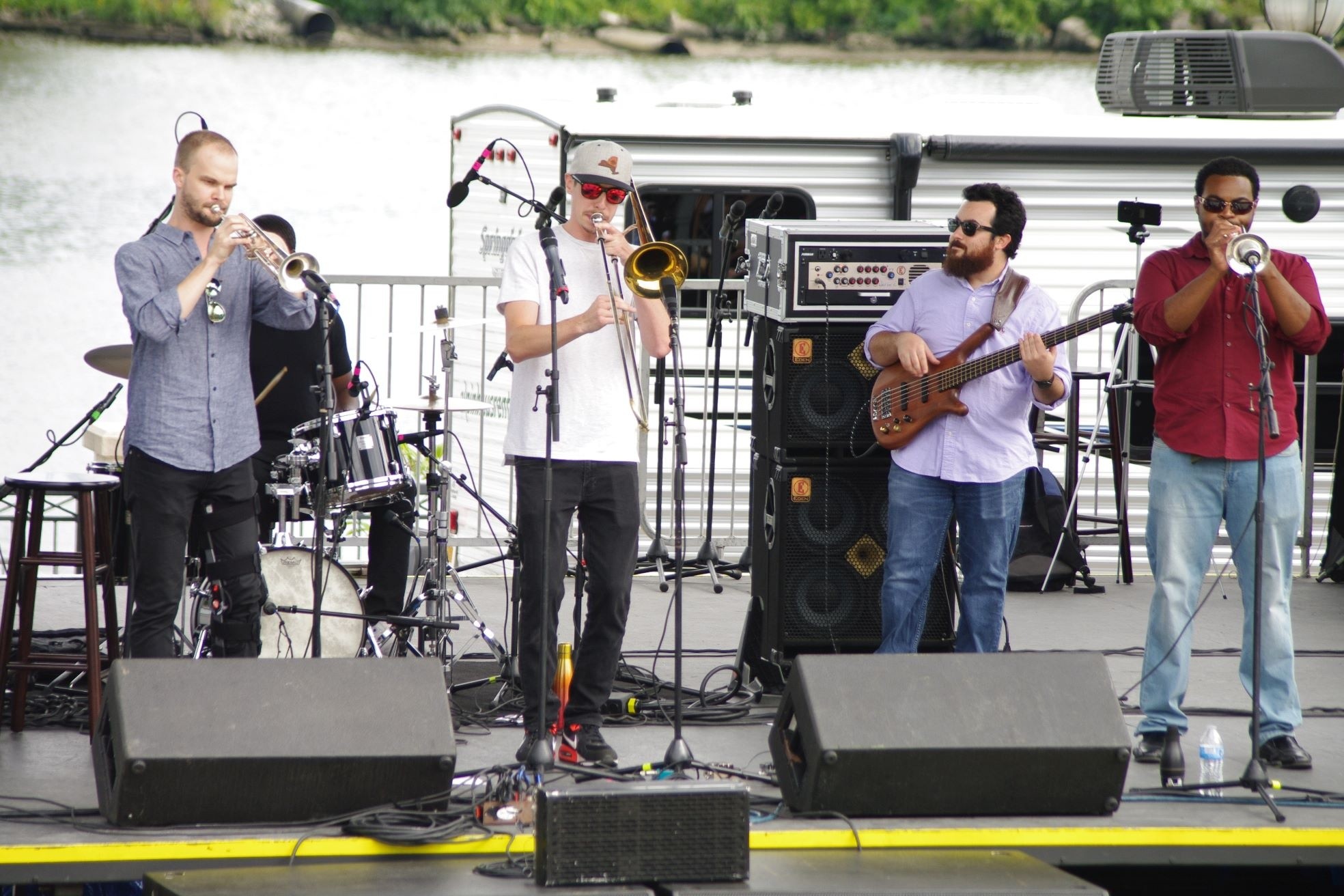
top-left (517, 722), bottom-right (557, 765)
top-left (561, 723), bottom-right (620, 766)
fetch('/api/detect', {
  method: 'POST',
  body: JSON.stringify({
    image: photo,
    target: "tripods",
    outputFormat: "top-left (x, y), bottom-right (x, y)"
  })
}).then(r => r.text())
top-left (1040, 317), bottom-right (1159, 593)
top-left (376, 433), bottom-right (514, 671)
top-left (1132, 267), bottom-right (1344, 819)
top-left (453, 172), bottom-right (783, 793)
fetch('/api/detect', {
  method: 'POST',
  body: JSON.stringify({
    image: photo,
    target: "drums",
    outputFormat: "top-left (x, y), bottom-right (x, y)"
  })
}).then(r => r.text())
top-left (82, 462), bottom-right (134, 586)
top-left (292, 407), bottom-right (413, 512)
top-left (189, 544), bottom-right (368, 659)
top-left (276, 453), bottom-right (320, 509)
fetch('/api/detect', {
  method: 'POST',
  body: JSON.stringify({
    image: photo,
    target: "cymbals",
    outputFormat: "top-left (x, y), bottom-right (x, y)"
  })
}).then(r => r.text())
top-left (388, 317), bottom-right (504, 338)
top-left (376, 396), bottom-right (495, 413)
top-left (83, 343), bottom-right (134, 380)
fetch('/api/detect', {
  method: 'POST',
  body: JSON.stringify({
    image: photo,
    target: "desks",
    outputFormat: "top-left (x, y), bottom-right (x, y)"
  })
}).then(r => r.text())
top-left (1044, 369), bottom-right (1134, 584)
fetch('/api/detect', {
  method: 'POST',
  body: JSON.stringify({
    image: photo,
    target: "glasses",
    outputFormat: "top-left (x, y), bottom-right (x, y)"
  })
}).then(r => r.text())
top-left (1198, 196), bottom-right (1257, 216)
top-left (571, 174), bottom-right (628, 204)
top-left (947, 217), bottom-right (998, 236)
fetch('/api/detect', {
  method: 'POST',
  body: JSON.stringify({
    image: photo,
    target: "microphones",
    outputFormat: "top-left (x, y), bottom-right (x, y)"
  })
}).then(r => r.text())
top-left (718, 200), bottom-right (746, 239)
top-left (447, 140), bottom-right (498, 208)
top-left (348, 361), bottom-right (361, 397)
top-left (539, 225), bottom-right (570, 304)
top-left (397, 429), bottom-right (446, 443)
top-left (758, 194), bottom-right (784, 219)
top-left (535, 188), bottom-right (564, 229)
top-left (386, 511), bottom-right (419, 539)
top-left (88, 383), bottom-right (123, 424)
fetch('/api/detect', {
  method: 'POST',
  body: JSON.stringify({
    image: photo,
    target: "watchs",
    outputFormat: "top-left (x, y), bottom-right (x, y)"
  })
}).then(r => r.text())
top-left (1034, 373), bottom-right (1055, 388)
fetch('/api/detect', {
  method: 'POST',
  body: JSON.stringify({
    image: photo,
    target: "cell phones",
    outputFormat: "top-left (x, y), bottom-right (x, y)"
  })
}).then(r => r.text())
top-left (1117, 200), bottom-right (1161, 227)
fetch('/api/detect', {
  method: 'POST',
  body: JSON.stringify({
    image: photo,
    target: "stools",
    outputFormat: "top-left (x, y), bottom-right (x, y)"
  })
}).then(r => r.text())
top-left (0, 473), bottom-right (126, 743)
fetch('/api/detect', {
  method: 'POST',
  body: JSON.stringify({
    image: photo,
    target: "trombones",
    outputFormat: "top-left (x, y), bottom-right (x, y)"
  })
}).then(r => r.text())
top-left (590, 178), bottom-right (690, 435)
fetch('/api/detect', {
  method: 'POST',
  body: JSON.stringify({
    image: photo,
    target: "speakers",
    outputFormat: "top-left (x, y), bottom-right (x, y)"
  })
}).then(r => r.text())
top-left (769, 654), bottom-right (1133, 813)
top-left (744, 455), bottom-right (959, 645)
top-left (1008, 468), bottom-right (1078, 592)
top-left (81, 654), bottom-right (458, 824)
top-left (750, 317), bottom-right (889, 456)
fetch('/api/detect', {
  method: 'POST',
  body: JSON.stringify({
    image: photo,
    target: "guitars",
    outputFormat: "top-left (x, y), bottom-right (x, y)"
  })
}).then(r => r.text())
top-left (870, 295), bottom-right (1137, 450)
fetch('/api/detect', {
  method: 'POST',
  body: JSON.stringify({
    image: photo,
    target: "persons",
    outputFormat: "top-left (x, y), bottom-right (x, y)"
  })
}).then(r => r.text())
top-left (114, 129), bottom-right (315, 658)
top-left (244, 214), bottom-right (422, 658)
top-left (497, 139), bottom-right (673, 765)
top-left (863, 184), bottom-right (1073, 653)
top-left (1132, 156), bottom-right (1332, 771)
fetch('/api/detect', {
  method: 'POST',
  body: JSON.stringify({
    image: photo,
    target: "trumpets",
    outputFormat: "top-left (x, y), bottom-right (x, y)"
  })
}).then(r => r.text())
top-left (211, 203), bottom-right (320, 294)
top-left (1223, 224), bottom-right (1271, 275)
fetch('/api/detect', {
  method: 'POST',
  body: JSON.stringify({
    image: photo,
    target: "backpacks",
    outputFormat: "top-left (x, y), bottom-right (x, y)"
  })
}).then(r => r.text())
top-left (1004, 465), bottom-right (1099, 591)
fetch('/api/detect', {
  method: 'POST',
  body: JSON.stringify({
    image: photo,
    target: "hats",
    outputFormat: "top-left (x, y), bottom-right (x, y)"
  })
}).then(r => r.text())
top-left (569, 138), bottom-right (636, 192)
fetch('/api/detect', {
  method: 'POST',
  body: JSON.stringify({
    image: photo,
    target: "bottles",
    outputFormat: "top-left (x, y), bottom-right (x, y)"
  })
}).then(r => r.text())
top-left (1199, 724), bottom-right (1223, 797)
top-left (1160, 726), bottom-right (1185, 787)
top-left (552, 644), bottom-right (574, 731)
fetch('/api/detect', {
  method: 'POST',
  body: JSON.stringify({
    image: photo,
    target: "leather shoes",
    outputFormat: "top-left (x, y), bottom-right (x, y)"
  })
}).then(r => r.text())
top-left (1260, 737), bottom-right (1316, 768)
top-left (1132, 731), bottom-right (1175, 762)
